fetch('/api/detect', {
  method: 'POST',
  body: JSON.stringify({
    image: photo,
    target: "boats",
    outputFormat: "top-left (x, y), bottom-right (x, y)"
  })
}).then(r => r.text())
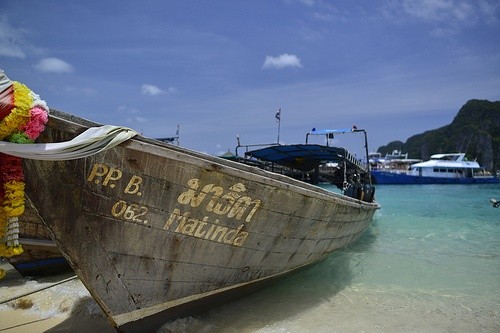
top-left (0, 69), bottom-right (381, 333)
top-left (360, 149), bottom-right (500, 184)
